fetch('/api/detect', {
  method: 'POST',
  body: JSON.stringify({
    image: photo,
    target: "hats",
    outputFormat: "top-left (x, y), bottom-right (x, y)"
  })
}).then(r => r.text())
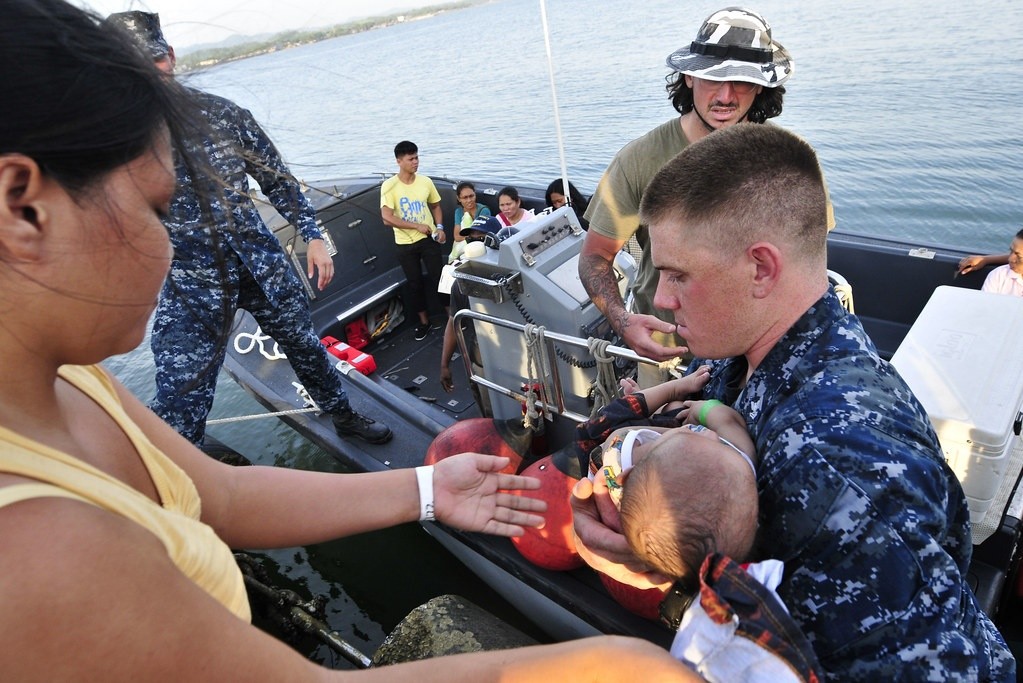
top-left (459, 215), bottom-right (502, 237)
top-left (666, 5), bottom-right (794, 88)
top-left (100, 11), bottom-right (170, 57)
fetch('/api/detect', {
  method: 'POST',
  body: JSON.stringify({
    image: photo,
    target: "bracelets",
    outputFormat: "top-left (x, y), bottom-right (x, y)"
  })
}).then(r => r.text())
top-left (699, 399), bottom-right (721, 425)
top-left (416, 466), bottom-right (436, 521)
top-left (436, 224), bottom-right (443, 229)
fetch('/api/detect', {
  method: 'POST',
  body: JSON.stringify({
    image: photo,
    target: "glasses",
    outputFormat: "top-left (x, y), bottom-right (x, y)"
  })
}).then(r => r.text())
top-left (459, 194), bottom-right (476, 199)
top-left (465, 236), bottom-right (485, 244)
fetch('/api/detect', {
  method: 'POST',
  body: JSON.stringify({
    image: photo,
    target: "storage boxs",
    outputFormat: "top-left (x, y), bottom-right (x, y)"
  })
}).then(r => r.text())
top-left (889, 285), bottom-right (1023, 524)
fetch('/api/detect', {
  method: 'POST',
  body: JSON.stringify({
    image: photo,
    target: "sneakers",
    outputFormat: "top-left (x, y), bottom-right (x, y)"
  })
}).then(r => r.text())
top-left (432, 321), bottom-right (446, 330)
top-left (414, 320), bottom-right (432, 341)
top-left (335, 410), bottom-right (392, 444)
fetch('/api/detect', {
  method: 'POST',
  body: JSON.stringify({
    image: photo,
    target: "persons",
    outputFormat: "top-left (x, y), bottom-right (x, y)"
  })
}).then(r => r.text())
top-left (958, 228), bottom-right (1023, 297)
top-left (579, 6), bottom-right (792, 394)
top-left (439, 217), bottom-right (505, 418)
top-left (495, 186), bottom-right (536, 228)
top-left (380, 141), bottom-right (446, 341)
top-left (571, 121), bottom-right (1016, 683)
top-left (97, 10), bottom-right (392, 454)
top-left (545, 178), bottom-right (590, 231)
top-left (453, 182), bottom-right (490, 241)
top-left (0, 0), bottom-right (706, 683)
top-left (592, 375), bottom-right (759, 578)
